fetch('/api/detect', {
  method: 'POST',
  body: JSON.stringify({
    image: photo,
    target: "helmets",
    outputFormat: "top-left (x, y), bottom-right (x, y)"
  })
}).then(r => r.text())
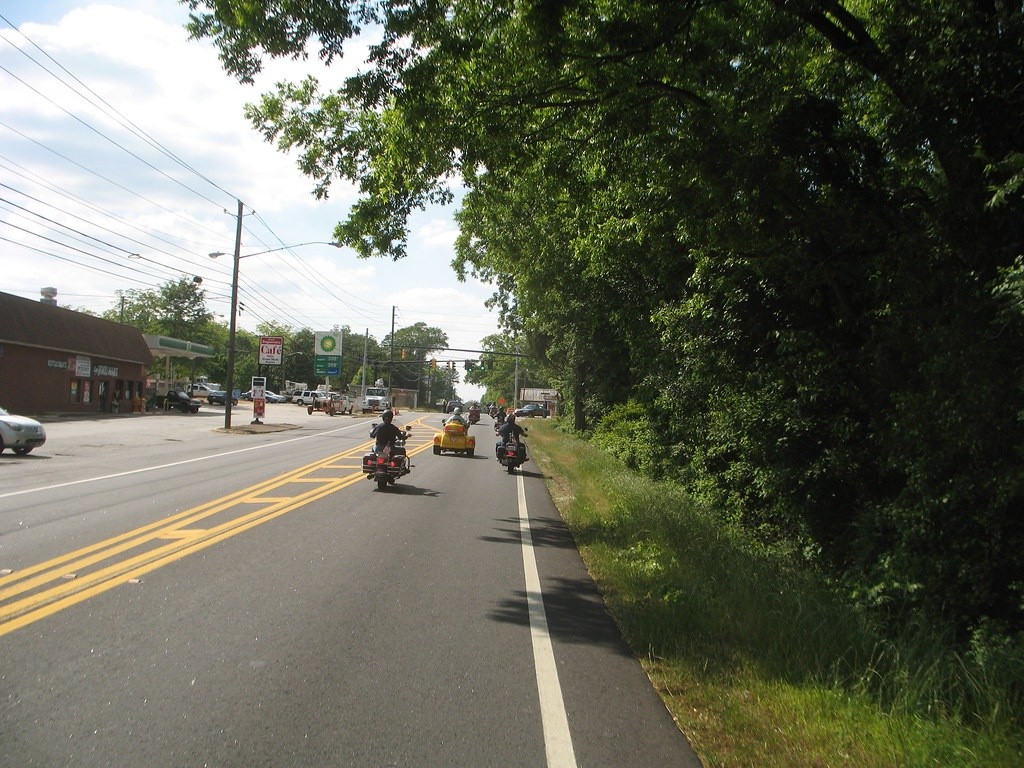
top-left (472, 401), bottom-right (476, 406)
top-left (492, 402), bottom-right (496, 406)
top-left (506, 413), bottom-right (515, 422)
top-left (499, 406), bottom-right (504, 412)
top-left (382, 409), bottom-right (394, 422)
top-left (454, 407), bottom-right (460, 415)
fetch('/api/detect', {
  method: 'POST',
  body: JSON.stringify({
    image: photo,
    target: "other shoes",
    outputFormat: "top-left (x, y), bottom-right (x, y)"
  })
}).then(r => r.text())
top-left (405, 468), bottom-right (410, 474)
top-left (524, 457), bottom-right (530, 461)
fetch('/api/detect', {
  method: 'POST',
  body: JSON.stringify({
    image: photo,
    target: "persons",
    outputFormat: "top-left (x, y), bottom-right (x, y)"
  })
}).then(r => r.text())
top-left (486, 403), bottom-right (496, 415)
top-left (496, 414), bottom-right (529, 461)
top-left (469, 403), bottom-right (480, 421)
top-left (369, 410), bottom-right (411, 476)
top-left (445, 408), bottom-right (470, 427)
top-left (494, 407), bottom-right (507, 428)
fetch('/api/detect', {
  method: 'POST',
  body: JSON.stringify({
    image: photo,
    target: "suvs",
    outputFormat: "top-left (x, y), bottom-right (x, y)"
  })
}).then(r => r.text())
top-left (186, 384), bottom-right (216, 399)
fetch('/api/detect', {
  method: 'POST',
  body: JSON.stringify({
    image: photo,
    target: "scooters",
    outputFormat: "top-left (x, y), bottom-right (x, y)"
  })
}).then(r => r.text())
top-left (432, 414), bottom-right (476, 457)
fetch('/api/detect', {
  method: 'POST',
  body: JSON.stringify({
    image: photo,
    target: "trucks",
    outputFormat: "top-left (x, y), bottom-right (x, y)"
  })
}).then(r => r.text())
top-left (147, 372), bottom-right (221, 397)
top-left (363, 388), bottom-right (391, 414)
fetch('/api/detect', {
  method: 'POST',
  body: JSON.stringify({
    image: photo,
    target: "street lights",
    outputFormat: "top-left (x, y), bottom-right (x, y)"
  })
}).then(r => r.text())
top-left (209, 242), bottom-right (343, 428)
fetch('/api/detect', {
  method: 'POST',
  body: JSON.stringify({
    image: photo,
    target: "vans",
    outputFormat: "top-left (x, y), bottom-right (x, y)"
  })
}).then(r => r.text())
top-left (291, 391), bottom-right (353, 420)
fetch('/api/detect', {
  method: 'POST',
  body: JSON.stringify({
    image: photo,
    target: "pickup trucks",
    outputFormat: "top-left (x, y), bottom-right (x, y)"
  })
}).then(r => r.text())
top-left (156, 390), bottom-right (200, 413)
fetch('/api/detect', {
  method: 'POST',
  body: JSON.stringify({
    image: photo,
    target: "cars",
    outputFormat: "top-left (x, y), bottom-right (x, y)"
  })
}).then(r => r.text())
top-left (242, 390), bottom-right (286, 404)
top-left (206, 390), bottom-right (238, 407)
top-left (513, 405), bottom-right (549, 418)
top-left (0, 408), bottom-right (46, 456)
top-left (446, 400), bottom-right (462, 413)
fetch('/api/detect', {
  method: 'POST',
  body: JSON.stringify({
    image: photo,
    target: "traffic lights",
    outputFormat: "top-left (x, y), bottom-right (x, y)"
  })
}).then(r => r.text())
top-left (480, 361), bottom-right (485, 370)
top-left (488, 359), bottom-right (493, 370)
top-left (446, 361), bottom-right (450, 373)
top-left (429, 359), bottom-right (435, 368)
top-left (400, 348), bottom-right (406, 360)
top-left (452, 361), bottom-right (455, 372)
top-left (468, 361), bottom-right (474, 372)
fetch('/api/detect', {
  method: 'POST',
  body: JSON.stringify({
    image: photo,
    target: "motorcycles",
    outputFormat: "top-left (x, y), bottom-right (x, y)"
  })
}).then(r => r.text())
top-left (486, 405), bottom-right (507, 429)
top-left (469, 408), bottom-right (482, 425)
top-left (495, 427), bottom-right (529, 475)
top-left (361, 419), bottom-right (415, 490)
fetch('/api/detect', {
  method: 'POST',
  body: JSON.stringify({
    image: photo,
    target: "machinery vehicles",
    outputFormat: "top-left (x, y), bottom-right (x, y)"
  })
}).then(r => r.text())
top-left (286, 380), bottom-right (311, 401)
